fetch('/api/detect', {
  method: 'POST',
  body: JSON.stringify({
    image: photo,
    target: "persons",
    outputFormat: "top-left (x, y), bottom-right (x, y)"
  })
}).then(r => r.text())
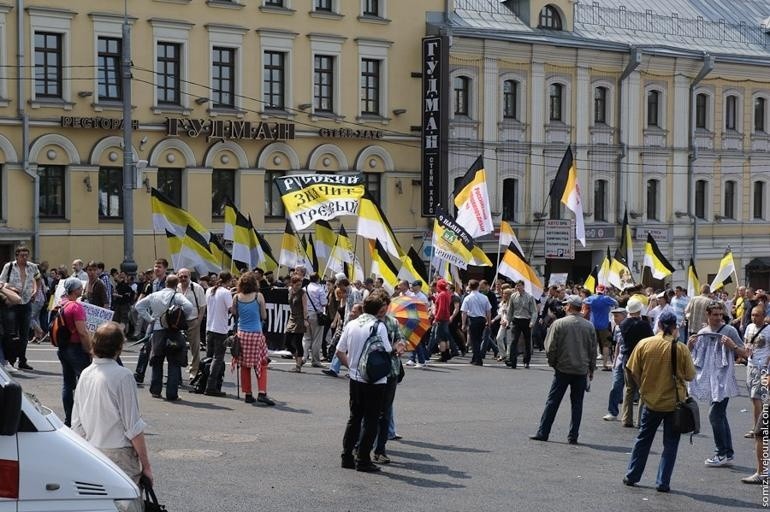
top-left (0, 248), bottom-right (769, 492)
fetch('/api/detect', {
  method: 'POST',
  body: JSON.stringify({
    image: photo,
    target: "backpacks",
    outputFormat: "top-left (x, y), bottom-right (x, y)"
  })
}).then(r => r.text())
top-left (47, 300), bottom-right (78, 347)
top-left (355, 319), bottom-right (392, 385)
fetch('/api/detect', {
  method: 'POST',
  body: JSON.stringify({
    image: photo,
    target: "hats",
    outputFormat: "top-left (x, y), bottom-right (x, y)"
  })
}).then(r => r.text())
top-left (611, 308), bottom-right (628, 316)
top-left (596, 284), bottom-right (607, 294)
top-left (625, 297), bottom-right (644, 313)
top-left (561, 294), bottom-right (583, 307)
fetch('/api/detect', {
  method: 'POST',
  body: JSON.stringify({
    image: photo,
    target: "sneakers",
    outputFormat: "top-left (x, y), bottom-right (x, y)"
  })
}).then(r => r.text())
top-left (529, 434), bottom-right (548, 442)
top-left (355, 460), bottom-right (381, 472)
top-left (245, 397), bottom-right (256, 404)
top-left (596, 352), bottom-right (615, 373)
top-left (389, 433), bottom-right (403, 441)
top-left (295, 356), bottom-right (339, 378)
top-left (134, 371), bottom-right (227, 403)
top-left (26, 332), bottom-right (49, 344)
top-left (741, 474), bottom-right (768, 484)
top-left (603, 414), bottom-right (617, 421)
top-left (5, 362), bottom-right (34, 373)
top-left (257, 396), bottom-right (276, 406)
top-left (744, 430), bottom-right (757, 438)
top-left (341, 455), bottom-right (356, 469)
top-left (656, 483), bottom-right (670, 493)
top-left (727, 454), bottom-right (736, 464)
top-left (568, 436), bottom-right (578, 445)
top-left (372, 451), bottom-right (391, 464)
top-left (704, 454), bottom-right (728, 466)
top-left (623, 474), bottom-right (635, 486)
top-left (406, 344), bottom-right (530, 369)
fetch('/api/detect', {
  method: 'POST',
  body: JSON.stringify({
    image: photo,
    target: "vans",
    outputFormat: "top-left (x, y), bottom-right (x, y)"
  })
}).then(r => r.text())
top-left (0, 359), bottom-right (147, 512)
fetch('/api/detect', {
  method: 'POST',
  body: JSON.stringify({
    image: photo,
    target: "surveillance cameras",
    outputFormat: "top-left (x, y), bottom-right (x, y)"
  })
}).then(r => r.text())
top-left (141, 136), bottom-right (148, 144)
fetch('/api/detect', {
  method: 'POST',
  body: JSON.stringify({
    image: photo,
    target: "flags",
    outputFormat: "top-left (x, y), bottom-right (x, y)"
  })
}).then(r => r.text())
top-left (150, 146), bottom-right (735, 299)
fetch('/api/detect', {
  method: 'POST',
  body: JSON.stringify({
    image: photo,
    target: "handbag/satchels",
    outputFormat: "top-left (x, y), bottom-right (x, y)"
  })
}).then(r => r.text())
top-left (160, 305), bottom-right (189, 331)
top-left (226, 335), bottom-right (240, 358)
top-left (672, 397), bottom-right (703, 434)
top-left (137, 473), bottom-right (168, 512)
top-left (316, 312), bottom-right (330, 326)
